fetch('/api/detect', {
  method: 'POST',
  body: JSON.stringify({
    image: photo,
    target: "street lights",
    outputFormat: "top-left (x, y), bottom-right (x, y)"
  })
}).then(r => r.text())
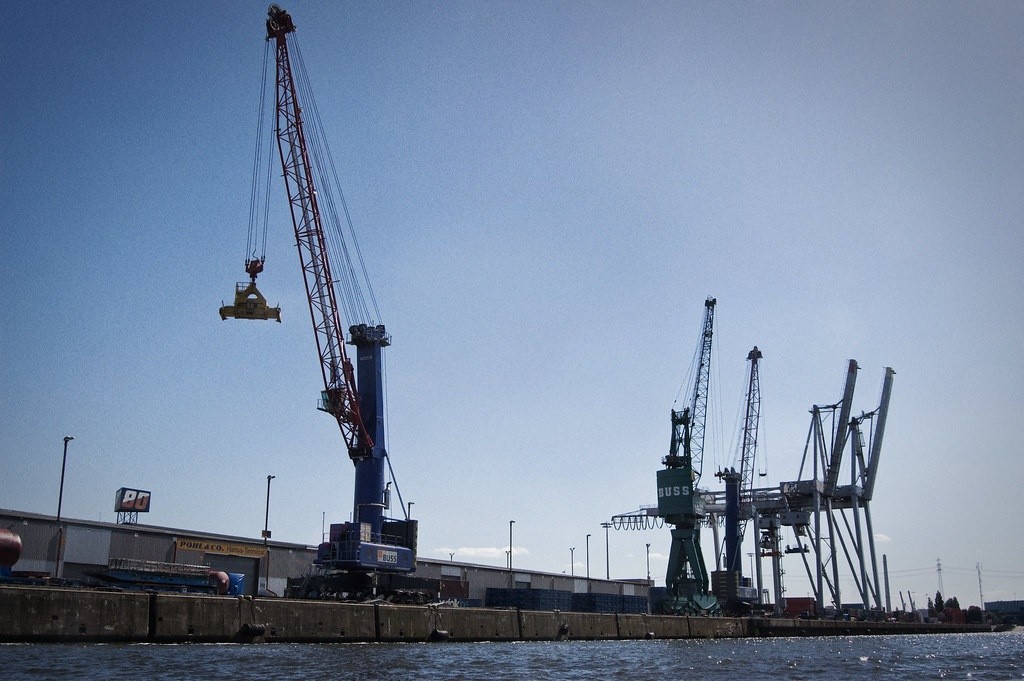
top-left (265, 475), bottom-right (278, 598)
top-left (54, 436), bottom-right (74, 577)
top-left (570, 546), bottom-right (575, 575)
top-left (586, 534), bottom-right (591, 577)
top-left (510, 520), bottom-right (516, 589)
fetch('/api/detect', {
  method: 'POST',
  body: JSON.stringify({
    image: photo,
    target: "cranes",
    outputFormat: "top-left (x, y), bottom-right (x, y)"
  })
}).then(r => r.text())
top-left (219, 2), bottom-right (442, 606)
top-left (647, 295), bottom-right (725, 619)
top-left (707, 345), bottom-right (763, 615)
